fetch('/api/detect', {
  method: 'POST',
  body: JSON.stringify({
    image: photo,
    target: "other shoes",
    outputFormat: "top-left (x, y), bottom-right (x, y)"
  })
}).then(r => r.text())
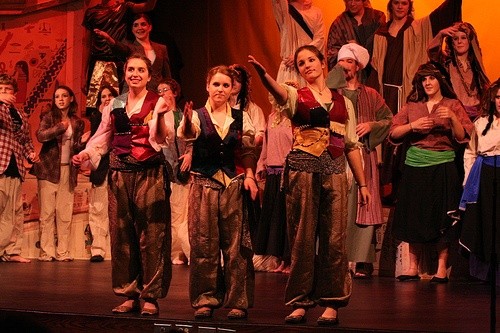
top-left (396, 273), bottom-right (420, 282)
top-left (431, 276), bottom-right (448, 284)
top-left (141, 301), bottom-right (160, 316)
top-left (112, 298), bottom-right (141, 314)
top-left (90, 254), bottom-right (105, 262)
top-left (354, 268), bottom-right (369, 278)
top-left (317, 306), bottom-right (339, 324)
top-left (227, 308), bottom-right (248, 319)
top-left (172, 258), bottom-right (186, 264)
top-left (284, 307), bottom-right (307, 323)
top-left (193, 307), bottom-right (214, 319)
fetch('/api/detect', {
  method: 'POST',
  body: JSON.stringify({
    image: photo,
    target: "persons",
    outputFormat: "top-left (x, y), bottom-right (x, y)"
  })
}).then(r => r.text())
top-left (84, 86), bottom-right (119, 263)
top-left (227, 64), bottom-right (299, 273)
top-left (370, 0), bottom-right (462, 116)
top-left (72, 54), bottom-right (176, 317)
top-left (249, 46), bottom-right (371, 323)
top-left (0, 73), bottom-right (40, 262)
top-left (94, 13), bottom-right (172, 95)
top-left (28, 85), bottom-right (91, 261)
top-left (157, 78), bottom-right (192, 264)
top-left (326, 0), bottom-right (386, 93)
top-left (324, 22), bottom-right (500, 287)
top-left (175, 66), bottom-right (259, 318)
top-left (82, 0), bottom-right (156, 90)
top-left (273, 0), bottom-right (327, 91)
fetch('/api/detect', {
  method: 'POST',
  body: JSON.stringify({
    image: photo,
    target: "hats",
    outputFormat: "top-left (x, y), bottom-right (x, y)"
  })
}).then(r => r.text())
top-left (338, 43), bottom-right (371, 69)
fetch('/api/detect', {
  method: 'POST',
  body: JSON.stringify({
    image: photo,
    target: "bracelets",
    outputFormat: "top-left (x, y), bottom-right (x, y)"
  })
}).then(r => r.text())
top-left (358, 184), bottom-right (367, 189)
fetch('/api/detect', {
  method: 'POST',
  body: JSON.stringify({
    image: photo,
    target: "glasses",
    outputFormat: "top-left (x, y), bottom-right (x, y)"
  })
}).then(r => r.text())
top-left (158, 87), bottom-right (171, 95)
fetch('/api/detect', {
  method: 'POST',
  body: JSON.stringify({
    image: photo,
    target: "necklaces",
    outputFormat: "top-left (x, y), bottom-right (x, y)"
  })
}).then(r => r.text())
top-left (307, 84), bottom-right (327, 95)
